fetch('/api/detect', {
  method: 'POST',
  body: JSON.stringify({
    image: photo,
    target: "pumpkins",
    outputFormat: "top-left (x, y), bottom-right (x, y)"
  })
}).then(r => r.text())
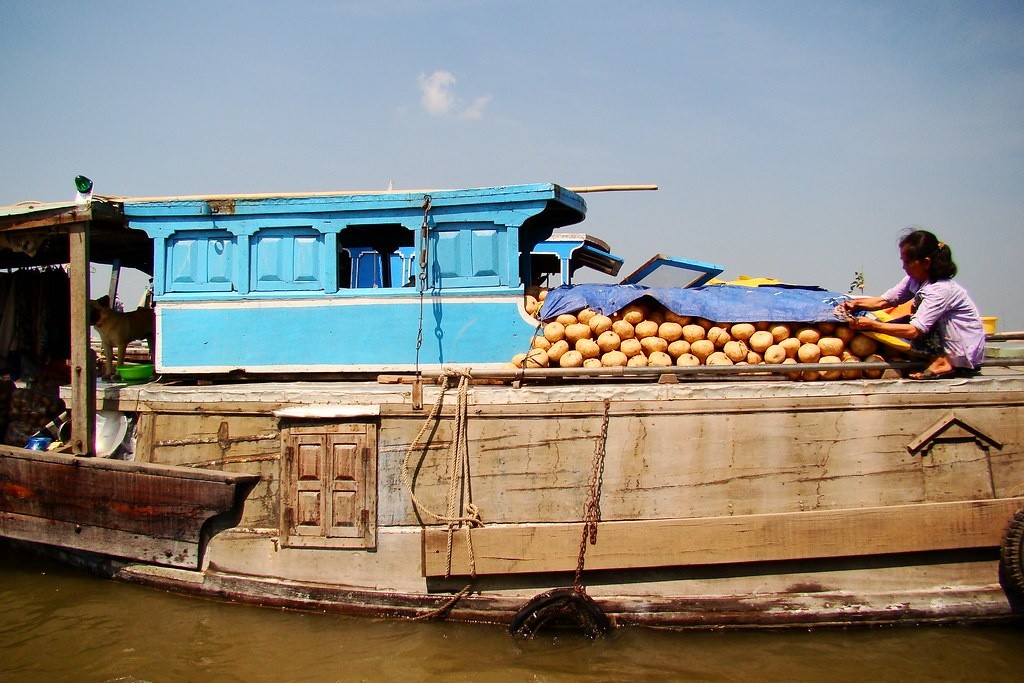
top-left (512, 283), bottom-right (907, 381)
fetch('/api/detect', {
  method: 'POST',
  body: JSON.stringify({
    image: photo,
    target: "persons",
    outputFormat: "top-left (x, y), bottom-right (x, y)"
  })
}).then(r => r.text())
top-left (403, 275), bottom-right (415, 287)
top-left (835, 227), bottom-right (986, 379)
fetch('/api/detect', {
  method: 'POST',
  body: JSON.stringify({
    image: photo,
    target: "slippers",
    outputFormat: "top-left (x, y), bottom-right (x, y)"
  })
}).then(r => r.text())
top-left (908, 366), bottom-right (956, 378)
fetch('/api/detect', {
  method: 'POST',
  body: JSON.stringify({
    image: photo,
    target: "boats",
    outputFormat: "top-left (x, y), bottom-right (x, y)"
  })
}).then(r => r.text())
top-left (0, 171), bottom-right (1024, 644)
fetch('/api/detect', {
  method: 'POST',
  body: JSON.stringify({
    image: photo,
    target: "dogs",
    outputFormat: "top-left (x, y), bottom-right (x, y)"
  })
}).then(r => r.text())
top-left (89, 295), bottom-right (155, 380)
